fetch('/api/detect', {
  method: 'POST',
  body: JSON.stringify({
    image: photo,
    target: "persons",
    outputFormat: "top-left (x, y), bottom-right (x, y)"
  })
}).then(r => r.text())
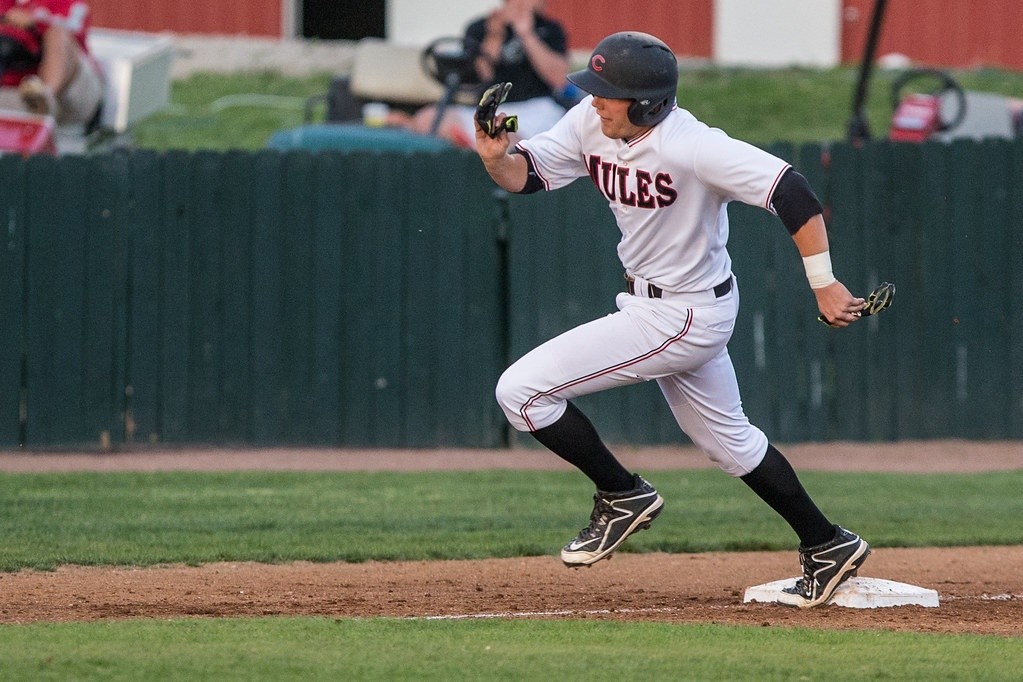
top-left (471, 29), bottom-right (869, 609)
top-left (404, 0), bottom-right (573, 147)
top-left (0, 0), bottom-right (107, 140)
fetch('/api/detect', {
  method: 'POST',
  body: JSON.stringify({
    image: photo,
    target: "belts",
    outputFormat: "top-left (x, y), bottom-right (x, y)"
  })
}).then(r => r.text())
top-left (623, 272), bottom-right (735, 298)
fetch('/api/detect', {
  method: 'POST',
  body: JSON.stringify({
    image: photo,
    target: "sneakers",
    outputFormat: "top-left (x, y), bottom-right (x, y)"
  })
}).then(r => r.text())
top-left (561, 473), bottom-right (664, 569)
top-left (778, 523), bottom-right (871, 611)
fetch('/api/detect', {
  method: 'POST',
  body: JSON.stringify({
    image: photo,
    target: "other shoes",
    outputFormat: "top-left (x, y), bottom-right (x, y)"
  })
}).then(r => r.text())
top-left (18, 75), bottom-right (52, 115)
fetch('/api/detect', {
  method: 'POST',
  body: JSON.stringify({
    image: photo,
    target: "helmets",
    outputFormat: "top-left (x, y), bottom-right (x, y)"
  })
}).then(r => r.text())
top-left (566, 31), bottom-right (679, 126)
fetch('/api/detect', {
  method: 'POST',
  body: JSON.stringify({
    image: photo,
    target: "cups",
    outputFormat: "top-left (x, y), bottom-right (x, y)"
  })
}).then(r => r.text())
top-left (362, 101), bottom-right (389, 128)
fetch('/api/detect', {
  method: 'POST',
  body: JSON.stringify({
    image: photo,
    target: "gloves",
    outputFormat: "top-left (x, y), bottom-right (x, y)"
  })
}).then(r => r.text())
top-left (817, 282), bottom-right (896, 329)
top-left (475, 82), bottom-right (518, 139)
